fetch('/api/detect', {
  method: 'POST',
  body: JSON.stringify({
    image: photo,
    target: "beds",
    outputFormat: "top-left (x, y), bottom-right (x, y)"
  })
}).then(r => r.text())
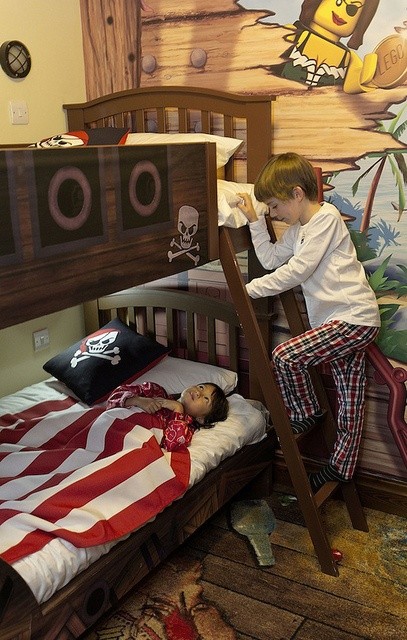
top-left (0, 85), bottom-right (277, 638)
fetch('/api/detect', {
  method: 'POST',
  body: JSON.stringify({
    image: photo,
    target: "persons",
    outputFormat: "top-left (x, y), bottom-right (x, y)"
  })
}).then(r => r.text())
top-left (226, 151), bottom-right (383, 501)
top-left (107, 379), bottom-right (230, 453)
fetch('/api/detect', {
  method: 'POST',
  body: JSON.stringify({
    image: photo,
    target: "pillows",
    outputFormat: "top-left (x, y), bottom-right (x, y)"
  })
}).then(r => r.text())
top-left (25, 127), bottom-right (131, 149)
top-left (126, 133), bottom-right (244, 171)
top-left (42, 318), bottom-right (169, 407)
top-left (47, 355), bottom-right (238, 401)
